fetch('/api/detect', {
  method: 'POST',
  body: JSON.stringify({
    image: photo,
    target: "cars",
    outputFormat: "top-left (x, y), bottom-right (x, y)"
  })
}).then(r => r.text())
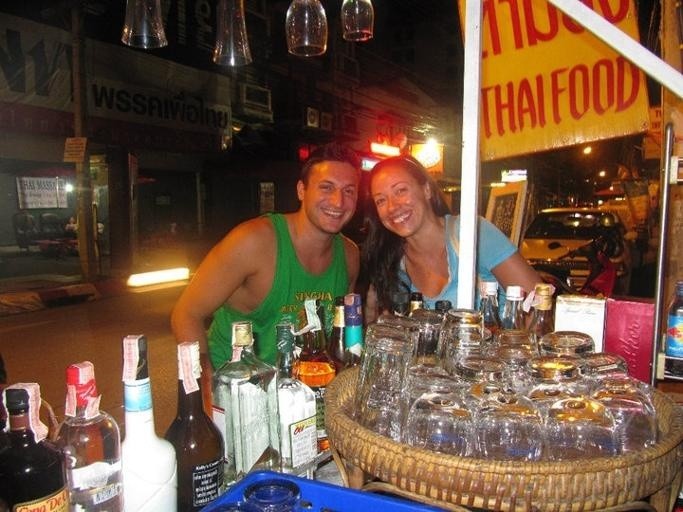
top-left (570, 161), bottom-right (659, 270)
top-left (513, 206), bottom-right (638, 295)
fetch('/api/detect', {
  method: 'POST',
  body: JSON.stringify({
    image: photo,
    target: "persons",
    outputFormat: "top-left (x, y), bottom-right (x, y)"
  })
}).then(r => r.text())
top-left (170, 144), bottom-right (360, 366)
top-left (364, 155), bottom-right (543, 328)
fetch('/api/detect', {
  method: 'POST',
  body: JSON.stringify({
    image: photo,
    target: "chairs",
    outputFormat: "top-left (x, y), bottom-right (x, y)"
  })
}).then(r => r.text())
top-left (13, 211), bottom-right (75, 257)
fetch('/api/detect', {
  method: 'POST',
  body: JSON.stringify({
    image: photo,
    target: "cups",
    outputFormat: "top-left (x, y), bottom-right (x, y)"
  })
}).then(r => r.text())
top-left (208, 1), bottom-right (251, 68)
top-left (353, 311), bottom-right (656, 460)
top-left (339, 1), bottom-right (375, 43)
top-left (284, 1), bottom-right (327, 57)
top-left (120, 0), bottom-right (168, 51)
top-left (243, 478), bottom-right (300, 511)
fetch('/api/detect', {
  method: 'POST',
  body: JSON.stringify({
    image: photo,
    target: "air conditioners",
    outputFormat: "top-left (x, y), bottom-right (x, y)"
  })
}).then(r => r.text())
top-left (319, 112), bottom-right (332, 131)
top-left (305, 106), bottom-right (320, 128)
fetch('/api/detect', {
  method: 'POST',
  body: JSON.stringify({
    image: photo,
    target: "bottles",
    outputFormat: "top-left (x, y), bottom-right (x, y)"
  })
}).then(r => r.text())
top-left (666, 279), bottom-right (683, 376)
top-left (121, 334), bottom-right (179, 512)
top-left (400, 282), bottom-right (555, 335)
top-left (166, 340), bottom-right (225, 508)
top-left (209, 320), bottom-right (283, 493)
top-left (0, 382), bottom-right (72, 512)
top-left (269, 291), bottom-right (366, 466)
top-left (56, 359), bottom-right (123, 512)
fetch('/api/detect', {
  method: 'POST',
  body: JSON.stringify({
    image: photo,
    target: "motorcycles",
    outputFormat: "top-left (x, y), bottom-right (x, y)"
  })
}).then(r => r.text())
top-left (531, 216), bottom-right (628, 297)
